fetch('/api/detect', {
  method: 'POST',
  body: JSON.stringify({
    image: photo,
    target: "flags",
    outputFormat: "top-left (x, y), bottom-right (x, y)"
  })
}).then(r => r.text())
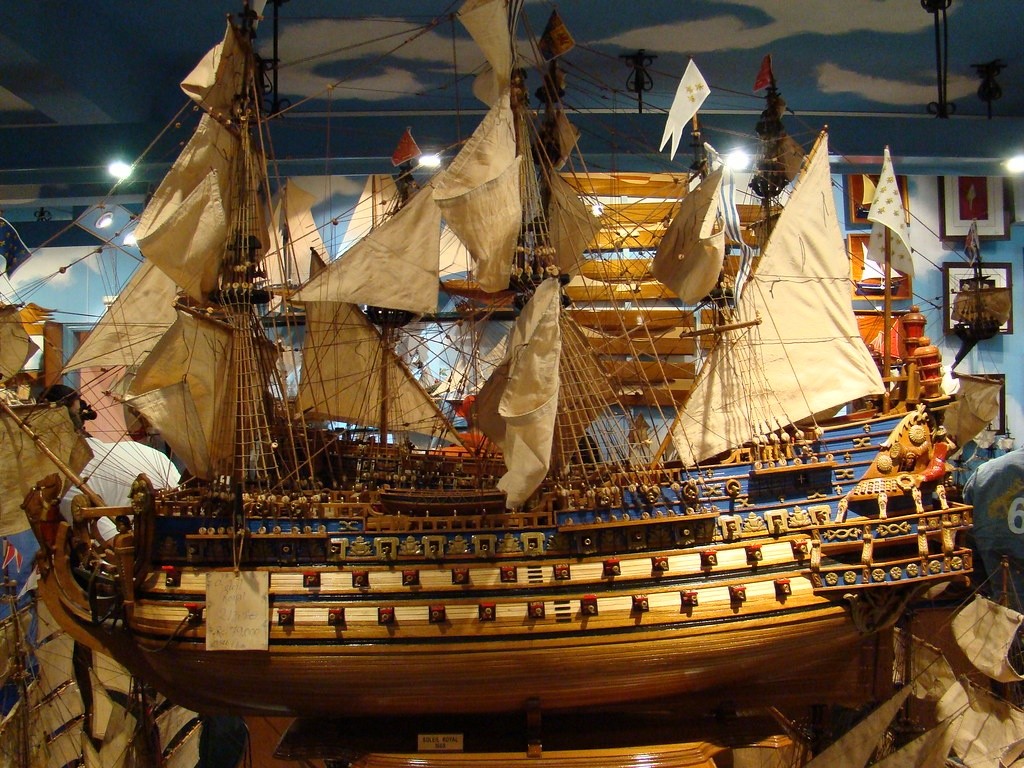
top-left (391, 127), bottom-right (422, 168)
top-left (754, 53), bottom-right (773, 93)
top-left (537, 9), bottom-right (576, 63)
top-left (964, 217), bottom-right (979, 267)
top-left (866, 144), bottom-right (916, 279)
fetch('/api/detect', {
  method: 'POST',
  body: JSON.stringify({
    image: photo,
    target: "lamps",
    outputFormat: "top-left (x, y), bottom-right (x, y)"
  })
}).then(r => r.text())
top-left (71, 201), bottom-right (146, 263)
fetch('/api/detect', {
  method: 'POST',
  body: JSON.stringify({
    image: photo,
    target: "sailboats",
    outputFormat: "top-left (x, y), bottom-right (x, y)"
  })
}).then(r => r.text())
top-left (1, 0), bottom-right (1022, 768)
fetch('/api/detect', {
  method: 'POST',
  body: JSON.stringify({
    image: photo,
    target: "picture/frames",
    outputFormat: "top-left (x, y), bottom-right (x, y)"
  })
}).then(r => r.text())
top-left (937, 174), bottom-right (1011, 241)
top-left (847, 233), bottom-right (912, 300)
top-left (846, 173), bottom-right (910, 225)
top-left (942, 262), bottom-right (1013, 335)
top-left (968, 374), bottom-right (1005, 436)
top-left (854, 310), bottom-right (911, 412)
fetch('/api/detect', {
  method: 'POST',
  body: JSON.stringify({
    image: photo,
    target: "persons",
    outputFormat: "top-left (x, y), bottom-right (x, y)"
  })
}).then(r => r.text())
top-left (58, 436), bottom-right (181, 548)
top-left (37, 384), bottom-right (81, 434)
